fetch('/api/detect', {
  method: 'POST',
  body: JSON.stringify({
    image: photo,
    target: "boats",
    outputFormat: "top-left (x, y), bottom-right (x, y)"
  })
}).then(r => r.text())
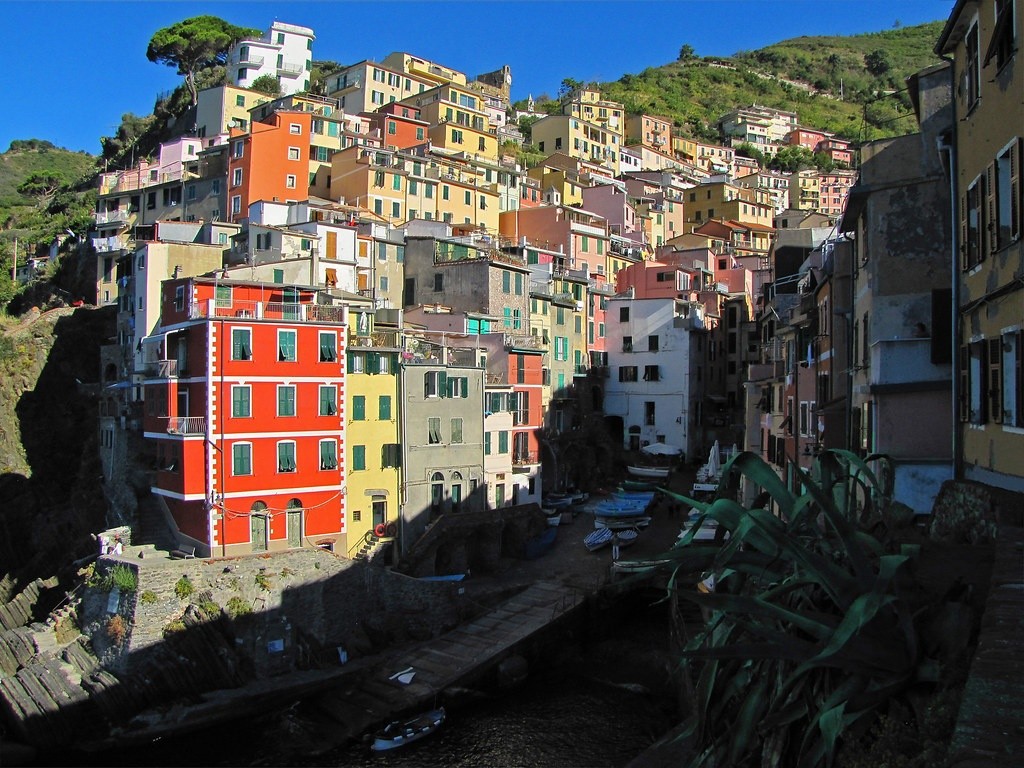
top-left (541, 490), bottom-right (589, 526)
top-left (610, 530), bottom-right (638, 547)
top-left (370, 707), bottom-right (446, 751)
top-left (584, 528), bottom-right (612, 551)
top-left (594, 491), bottom-right (656, 516)
top-left (594, 517), bottom-right (651, 529)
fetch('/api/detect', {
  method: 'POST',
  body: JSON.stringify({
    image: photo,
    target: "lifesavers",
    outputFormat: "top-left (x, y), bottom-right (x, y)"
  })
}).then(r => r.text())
top-left (375, 524), bottom-right (386, 536)
top-left (385, 521), bottom-right (397, 536)
top-left (365, 531), bottom-right (376, 545)
